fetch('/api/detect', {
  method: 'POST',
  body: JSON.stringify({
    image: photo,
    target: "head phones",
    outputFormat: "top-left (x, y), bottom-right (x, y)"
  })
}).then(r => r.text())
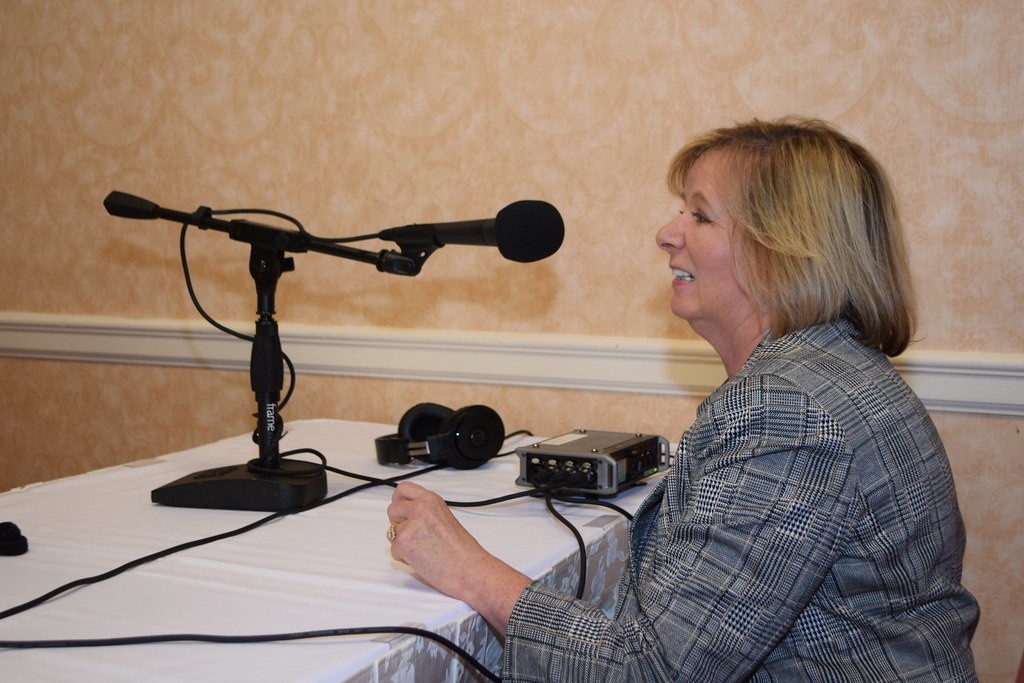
top-left (374, 402), bottom-right (505, 470)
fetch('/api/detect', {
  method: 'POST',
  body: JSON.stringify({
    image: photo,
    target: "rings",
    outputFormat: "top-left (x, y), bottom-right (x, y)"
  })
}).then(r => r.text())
top-left (388, 522), bottom-right (397, 540)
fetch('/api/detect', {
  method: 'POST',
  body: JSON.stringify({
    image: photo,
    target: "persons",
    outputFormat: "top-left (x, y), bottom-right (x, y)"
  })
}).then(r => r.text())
top-left (387, 117), bottom-right (981, 683)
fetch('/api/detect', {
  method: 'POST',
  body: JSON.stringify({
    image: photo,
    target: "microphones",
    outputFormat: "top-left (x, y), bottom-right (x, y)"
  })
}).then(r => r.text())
top-left (378, 200), bottom-right (565, 263)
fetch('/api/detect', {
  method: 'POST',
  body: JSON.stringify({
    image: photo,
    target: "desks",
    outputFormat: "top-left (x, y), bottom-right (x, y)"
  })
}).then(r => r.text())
top-left (0, 419), bottom-right (679, 683)
